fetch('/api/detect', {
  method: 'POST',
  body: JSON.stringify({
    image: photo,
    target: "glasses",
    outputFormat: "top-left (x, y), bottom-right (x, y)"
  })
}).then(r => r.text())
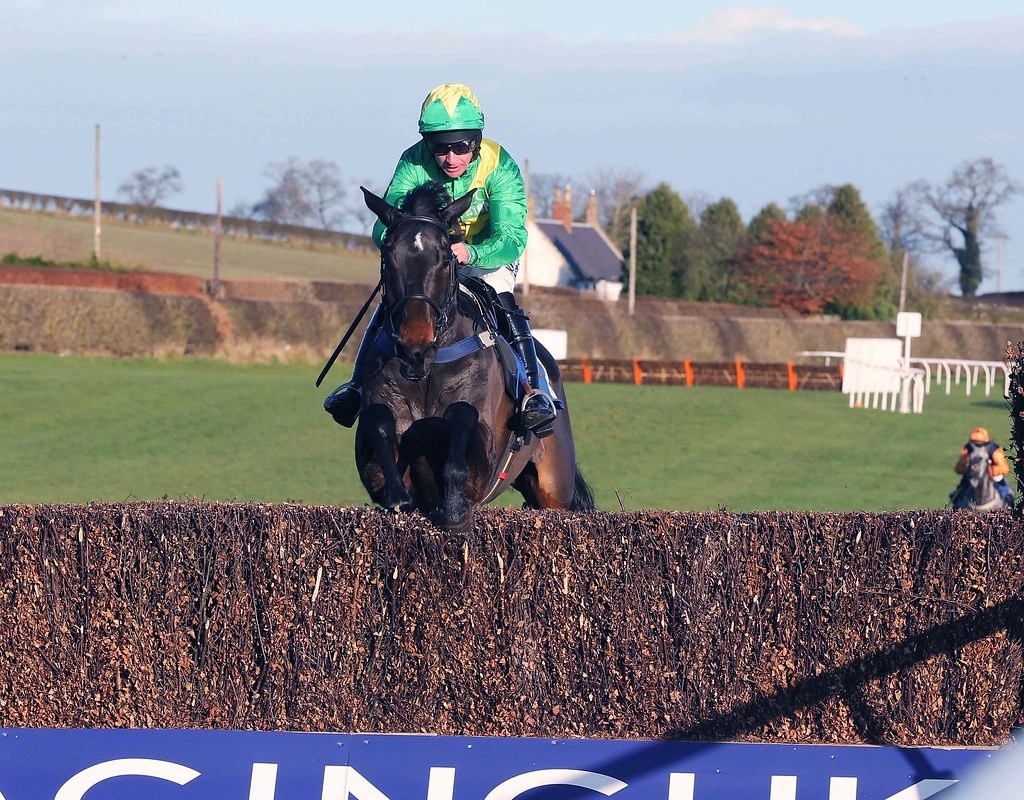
top-left (429, 138), bottom-right (476, 157)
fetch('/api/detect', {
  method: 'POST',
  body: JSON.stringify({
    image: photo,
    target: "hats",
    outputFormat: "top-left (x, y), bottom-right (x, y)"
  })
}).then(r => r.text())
top-left (416, 83), bottom-right (485, 132)
top-left (969, 428), bottom-right (992, 444)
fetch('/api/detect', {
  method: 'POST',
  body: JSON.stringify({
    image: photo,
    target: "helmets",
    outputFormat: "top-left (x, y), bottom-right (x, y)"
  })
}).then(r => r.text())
top-left (424, 128), bottom-right (482, 160)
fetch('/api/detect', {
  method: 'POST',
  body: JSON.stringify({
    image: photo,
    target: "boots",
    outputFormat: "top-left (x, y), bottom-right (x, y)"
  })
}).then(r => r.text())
top-left (496, 307), bottom-right (556, 441)
top-left (324, 304), bottom-right (394, 428)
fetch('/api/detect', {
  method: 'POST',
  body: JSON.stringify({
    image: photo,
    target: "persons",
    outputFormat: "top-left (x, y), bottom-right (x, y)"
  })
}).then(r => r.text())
top-left (949, 426), bottom-right (1015, 512)
top-left (323, 82), bottom-right (557, 439)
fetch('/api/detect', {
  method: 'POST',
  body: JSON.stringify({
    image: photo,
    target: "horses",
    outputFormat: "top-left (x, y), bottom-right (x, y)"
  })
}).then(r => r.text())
top-left (957, 440), bottom-right (1010, 510)
top-left (355, 182), bottom-right (596, 535)
